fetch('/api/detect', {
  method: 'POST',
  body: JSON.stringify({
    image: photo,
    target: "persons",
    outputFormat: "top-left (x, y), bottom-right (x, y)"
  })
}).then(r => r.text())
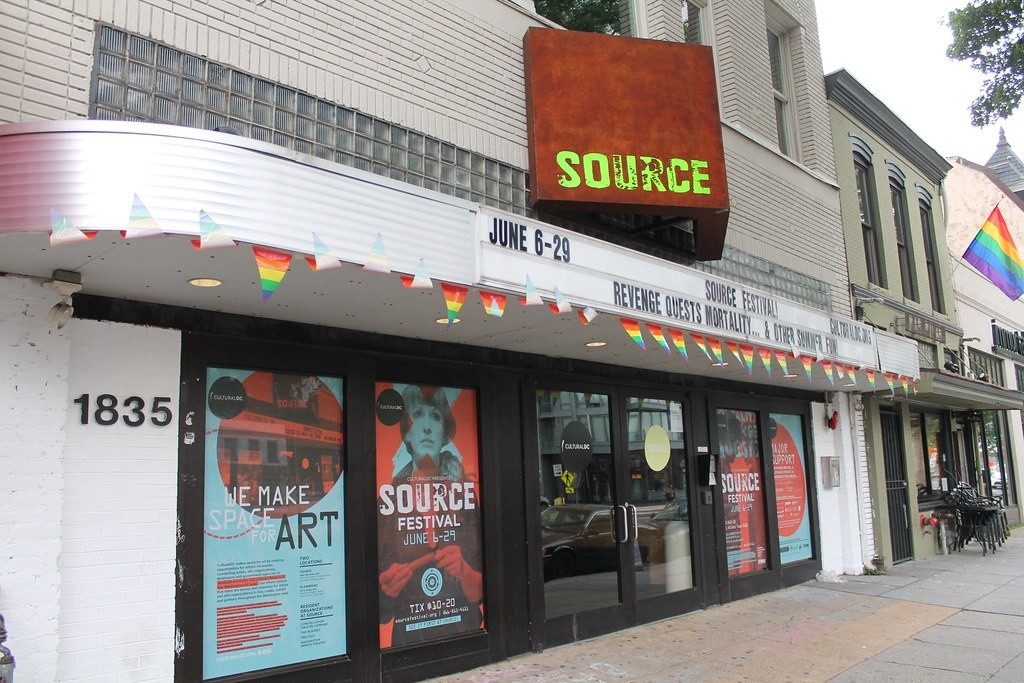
top-left (377, 383), bottom-right (485, 647)
top-left (664, 484), bottom-right (675, 502)
top-left (591, 473), bottom-right (602, 503)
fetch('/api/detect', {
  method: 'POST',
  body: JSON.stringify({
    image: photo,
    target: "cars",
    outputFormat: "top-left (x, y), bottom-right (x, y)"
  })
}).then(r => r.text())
top-left (541, 504), bottom-right (662, 580)
top-left (990, 464), bottom-right (1008, 488)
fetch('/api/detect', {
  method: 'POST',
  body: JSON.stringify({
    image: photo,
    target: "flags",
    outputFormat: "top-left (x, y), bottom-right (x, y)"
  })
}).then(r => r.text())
top-left (961, 205), bottom-right (1024, 301)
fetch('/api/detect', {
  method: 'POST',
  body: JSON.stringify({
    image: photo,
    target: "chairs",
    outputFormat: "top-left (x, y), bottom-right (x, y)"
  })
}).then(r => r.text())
top-left (944, 481), bottom-right (1012, 558)
